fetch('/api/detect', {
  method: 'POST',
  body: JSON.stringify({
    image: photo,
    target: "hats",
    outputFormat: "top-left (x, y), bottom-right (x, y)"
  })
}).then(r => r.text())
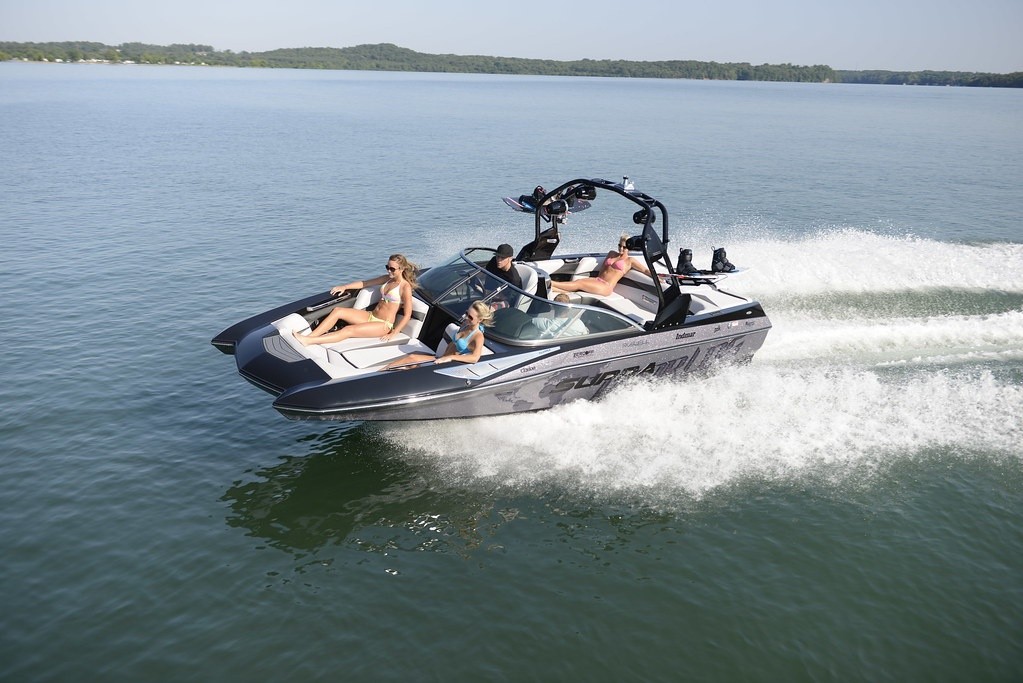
top-left (493, 244), bottom-right (513, 257)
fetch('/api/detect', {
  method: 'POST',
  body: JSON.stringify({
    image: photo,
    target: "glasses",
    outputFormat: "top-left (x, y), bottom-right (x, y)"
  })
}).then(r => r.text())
top-left (385, 265), bottom-right (398, 272)
top-left (618, 244), bottom-right (626, 249)
top-left (465, 310), bottom-right (473, 321)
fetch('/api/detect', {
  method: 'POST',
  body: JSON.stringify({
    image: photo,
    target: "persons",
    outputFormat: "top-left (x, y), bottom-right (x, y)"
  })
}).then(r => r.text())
top-left (551, 232), bottom-right (666, 298)
top-left (377, 300), bottom-right (489, 372)
top-left (532, 294), bottom-right (590, 339)
top-left (293, 254), bottom-right (413, 346)
top-left (471, 243), bottom-right (523, 313)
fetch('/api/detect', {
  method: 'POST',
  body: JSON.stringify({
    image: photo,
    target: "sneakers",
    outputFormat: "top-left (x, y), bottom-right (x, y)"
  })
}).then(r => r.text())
top-left (676, 248), bottom-right (699, 275)
top-left (711, 246), bottom-right (739, 272)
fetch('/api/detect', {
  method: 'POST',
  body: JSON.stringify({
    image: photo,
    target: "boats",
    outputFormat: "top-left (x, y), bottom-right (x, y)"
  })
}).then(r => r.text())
top-left (211, 176), bottom-right (772, 422)
